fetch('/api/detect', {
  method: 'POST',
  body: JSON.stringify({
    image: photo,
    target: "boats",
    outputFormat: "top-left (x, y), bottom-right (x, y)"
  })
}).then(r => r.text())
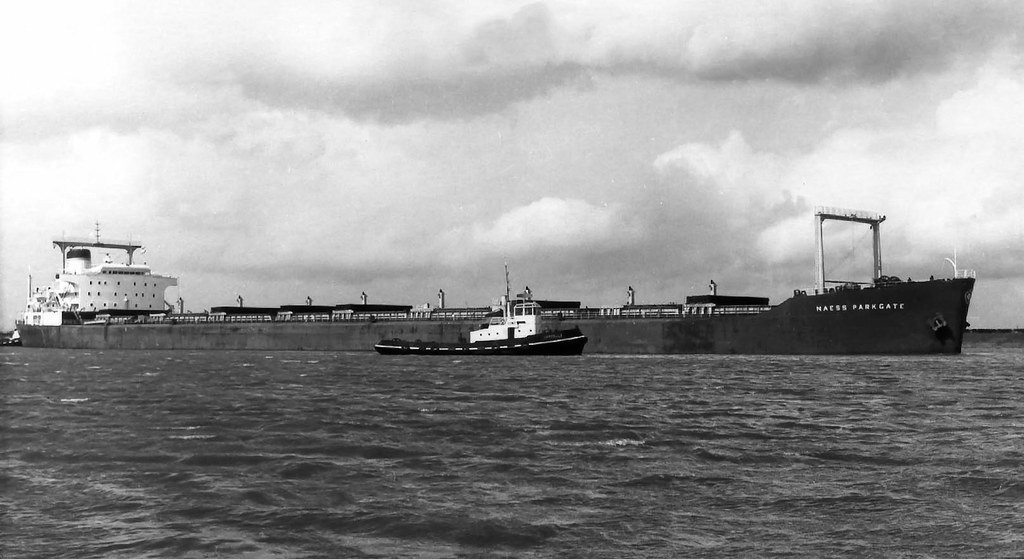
top-left (373, 265), bottom-right (587, 358)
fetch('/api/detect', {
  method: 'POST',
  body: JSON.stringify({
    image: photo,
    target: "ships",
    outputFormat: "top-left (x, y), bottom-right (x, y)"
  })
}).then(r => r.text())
top-left (13, 207), bottom-right (979, 357)
